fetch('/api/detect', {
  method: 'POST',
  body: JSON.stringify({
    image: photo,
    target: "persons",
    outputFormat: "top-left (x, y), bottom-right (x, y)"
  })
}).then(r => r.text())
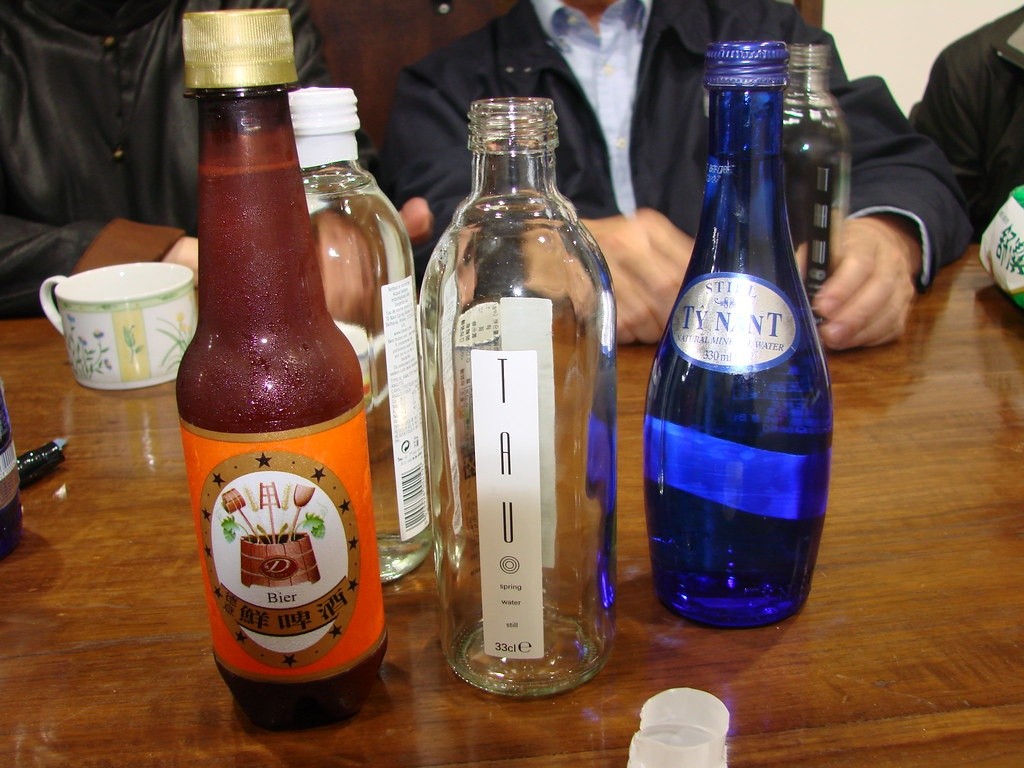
top-left (380, 0), bottom-right (974, 350)
top-left (909, 0), bottom-right (1024, 244)
top-left (0, 0), bottom-right (435, 328)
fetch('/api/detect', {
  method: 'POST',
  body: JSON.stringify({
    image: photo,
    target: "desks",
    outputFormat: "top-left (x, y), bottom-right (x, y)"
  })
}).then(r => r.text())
top-left (0, 244), bottom-right (1024, 768)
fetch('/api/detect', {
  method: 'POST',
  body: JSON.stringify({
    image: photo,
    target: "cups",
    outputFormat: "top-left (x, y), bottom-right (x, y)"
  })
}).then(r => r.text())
top-left (40, 262), bottom-right (198, 390)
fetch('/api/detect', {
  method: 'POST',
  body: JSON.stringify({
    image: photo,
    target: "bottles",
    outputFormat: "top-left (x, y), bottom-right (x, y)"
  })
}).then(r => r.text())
top-left (173, 7), bottom-right (388, 731)
top-left (285, 86), bottom-right (432, 582)
top-left (643, 36), bottom-right (835, 628)
top-left (779, 42), bottom-right (847, 356)
top-left (420, 98), bottom-right (618, 698)
